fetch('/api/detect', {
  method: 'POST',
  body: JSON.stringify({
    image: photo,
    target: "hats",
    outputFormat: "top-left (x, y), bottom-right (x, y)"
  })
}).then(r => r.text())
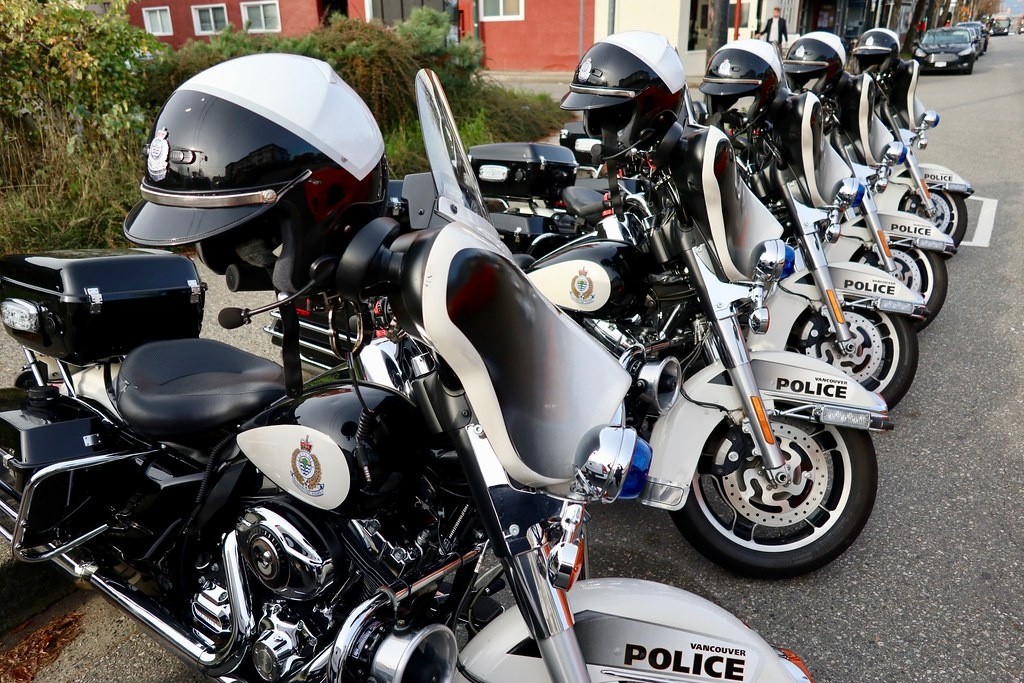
top-left (920, 18), bottom-right (927, 22)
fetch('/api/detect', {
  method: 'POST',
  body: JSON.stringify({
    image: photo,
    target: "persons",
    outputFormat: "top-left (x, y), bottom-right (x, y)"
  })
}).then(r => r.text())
top-left (754, 7), bottom-right (788, 58)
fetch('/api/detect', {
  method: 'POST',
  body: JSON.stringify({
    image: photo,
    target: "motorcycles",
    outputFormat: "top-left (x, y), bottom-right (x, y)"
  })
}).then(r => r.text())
top-left (1, 30), bottom-right (975, 683)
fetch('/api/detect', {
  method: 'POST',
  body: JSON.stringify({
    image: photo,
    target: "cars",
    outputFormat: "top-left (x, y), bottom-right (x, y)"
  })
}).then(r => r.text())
top-left (910, 27), bottom-right (977, 77)
top-left (955, 21), bottom-right (990, 57)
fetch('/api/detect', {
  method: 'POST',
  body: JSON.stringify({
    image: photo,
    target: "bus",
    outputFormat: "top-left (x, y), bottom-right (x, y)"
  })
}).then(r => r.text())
top-left (990, 17), bottom-right (1011, 35)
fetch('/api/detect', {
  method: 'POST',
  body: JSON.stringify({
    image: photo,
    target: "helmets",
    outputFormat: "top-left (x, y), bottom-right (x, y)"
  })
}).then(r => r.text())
top-left (697, 39), bottom-right (783, 128)
top-left (784, 32), bottom-right (845, 92)
top-left (852, 27), bottom-right (901, 71)
top-left (117, 52), bottom-right (386, 282)
top-left (559, 32), bottom-right (688, 152)
